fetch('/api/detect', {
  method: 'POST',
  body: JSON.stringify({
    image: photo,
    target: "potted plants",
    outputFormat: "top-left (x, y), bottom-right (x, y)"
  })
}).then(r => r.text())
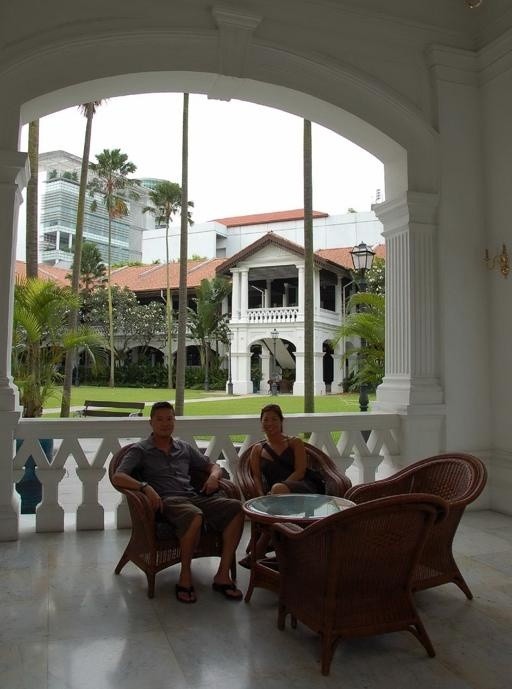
top-left (13, 274), bottom-right (110, 514)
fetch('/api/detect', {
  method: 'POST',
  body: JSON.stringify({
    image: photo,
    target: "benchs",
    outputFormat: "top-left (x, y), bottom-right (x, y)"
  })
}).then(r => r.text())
top-left (77, 399), bottom-right (145, 418)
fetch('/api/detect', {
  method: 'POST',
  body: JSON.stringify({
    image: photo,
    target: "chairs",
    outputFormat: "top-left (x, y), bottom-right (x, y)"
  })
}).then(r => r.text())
top-left (271, 492), bottom-right (451, 677)
top-left (233, 437), bottom-right (354, 561)
top-left (109, 437), bottom-right (245, 600)
top-left (342, 450), bottom-right (487, 604)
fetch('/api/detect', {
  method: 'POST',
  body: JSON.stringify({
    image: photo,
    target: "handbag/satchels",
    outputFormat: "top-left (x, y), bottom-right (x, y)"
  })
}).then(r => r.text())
top-left (304, 467), bottom-right (326, 494)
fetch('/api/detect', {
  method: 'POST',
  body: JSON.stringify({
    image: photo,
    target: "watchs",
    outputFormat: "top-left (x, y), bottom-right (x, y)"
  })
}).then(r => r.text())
top-left (140, 482), bottom-right (147, 491)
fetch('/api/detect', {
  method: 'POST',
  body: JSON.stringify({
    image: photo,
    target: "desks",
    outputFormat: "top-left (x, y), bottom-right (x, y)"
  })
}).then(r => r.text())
top-left (243, 492), bottom-right (358, 605)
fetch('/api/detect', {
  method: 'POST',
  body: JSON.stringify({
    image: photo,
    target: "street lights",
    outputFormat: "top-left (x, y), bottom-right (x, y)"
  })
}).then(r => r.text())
top-left (349, 240), bottom-right (376, 422)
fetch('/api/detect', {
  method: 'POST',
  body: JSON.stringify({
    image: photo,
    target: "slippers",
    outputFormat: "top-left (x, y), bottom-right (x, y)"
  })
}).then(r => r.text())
top-left (175, 583), bottom-right (197, 603)
top-left (212, 582), bottom-right (243, 601)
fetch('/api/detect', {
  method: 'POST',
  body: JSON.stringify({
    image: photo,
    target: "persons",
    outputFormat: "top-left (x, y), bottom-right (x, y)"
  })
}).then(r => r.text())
top-left (112, 401), bottom-right (244, 604)
top-left (236, 403), bottom-right (313, 570)
